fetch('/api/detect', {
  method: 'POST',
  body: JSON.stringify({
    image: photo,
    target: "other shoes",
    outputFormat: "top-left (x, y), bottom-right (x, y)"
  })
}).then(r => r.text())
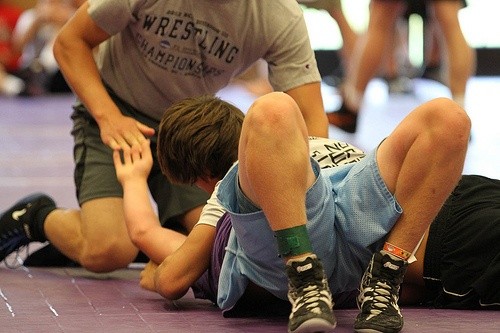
top-left (0, 192), bottom-right (56, 269)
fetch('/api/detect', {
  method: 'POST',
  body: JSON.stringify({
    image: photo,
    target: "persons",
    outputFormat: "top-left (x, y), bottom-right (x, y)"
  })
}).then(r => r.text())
top-left (112, 90), bottom-right (471, 333)
top-left (139, 96), bottom-right (499, 310)
top-left (0, 0), bottom-right (477, 143)
top-left (0, 0), bottom-right (329, 275)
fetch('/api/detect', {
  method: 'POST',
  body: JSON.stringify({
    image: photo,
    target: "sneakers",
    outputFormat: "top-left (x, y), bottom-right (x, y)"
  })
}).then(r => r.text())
top-left (285, 253), bottom-right (337, 332)
top-left (353, 249), bottom-right (404, 332)
